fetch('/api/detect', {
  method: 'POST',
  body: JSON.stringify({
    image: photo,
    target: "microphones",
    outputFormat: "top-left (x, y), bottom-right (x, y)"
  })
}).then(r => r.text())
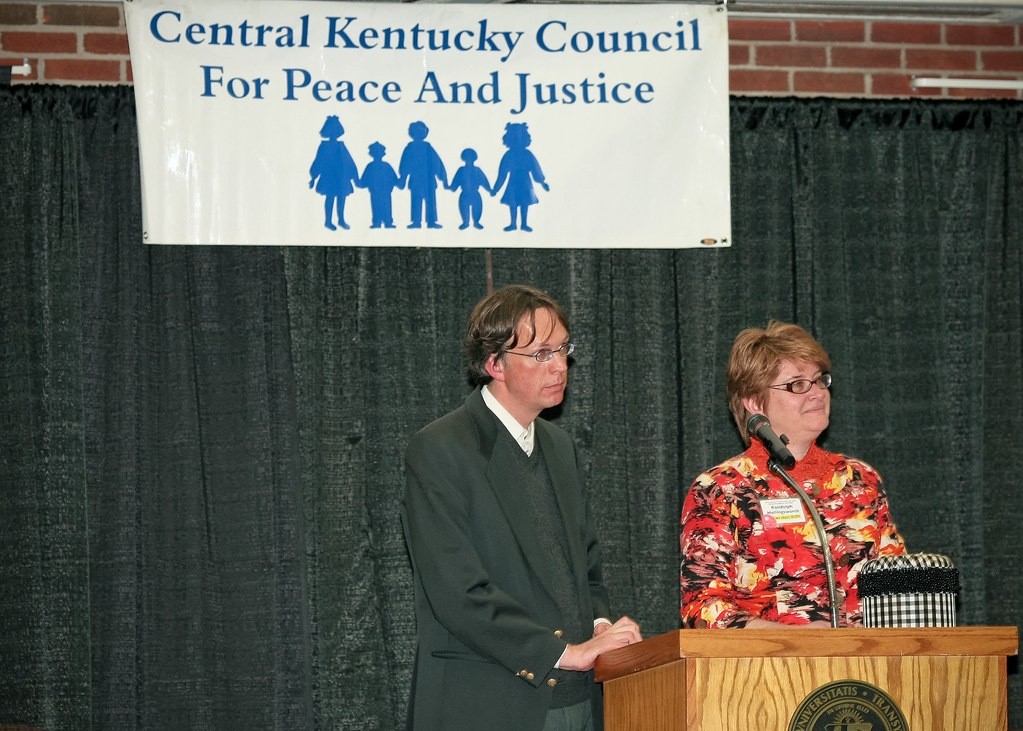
top-left (746, 414), bottom-right (796, 471)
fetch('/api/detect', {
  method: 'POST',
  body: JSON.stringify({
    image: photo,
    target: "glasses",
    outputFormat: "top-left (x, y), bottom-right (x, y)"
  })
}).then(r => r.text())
top-left (767, 374), bottom-right (832, 395)
top-left (505, 343), bottom-right (575, 362)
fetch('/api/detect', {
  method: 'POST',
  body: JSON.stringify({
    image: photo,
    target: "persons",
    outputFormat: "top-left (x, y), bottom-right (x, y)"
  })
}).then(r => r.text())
top-left (680, 319), bottom-right (907, 629)
top-left (401, 284), bottom-right (642, 731)
